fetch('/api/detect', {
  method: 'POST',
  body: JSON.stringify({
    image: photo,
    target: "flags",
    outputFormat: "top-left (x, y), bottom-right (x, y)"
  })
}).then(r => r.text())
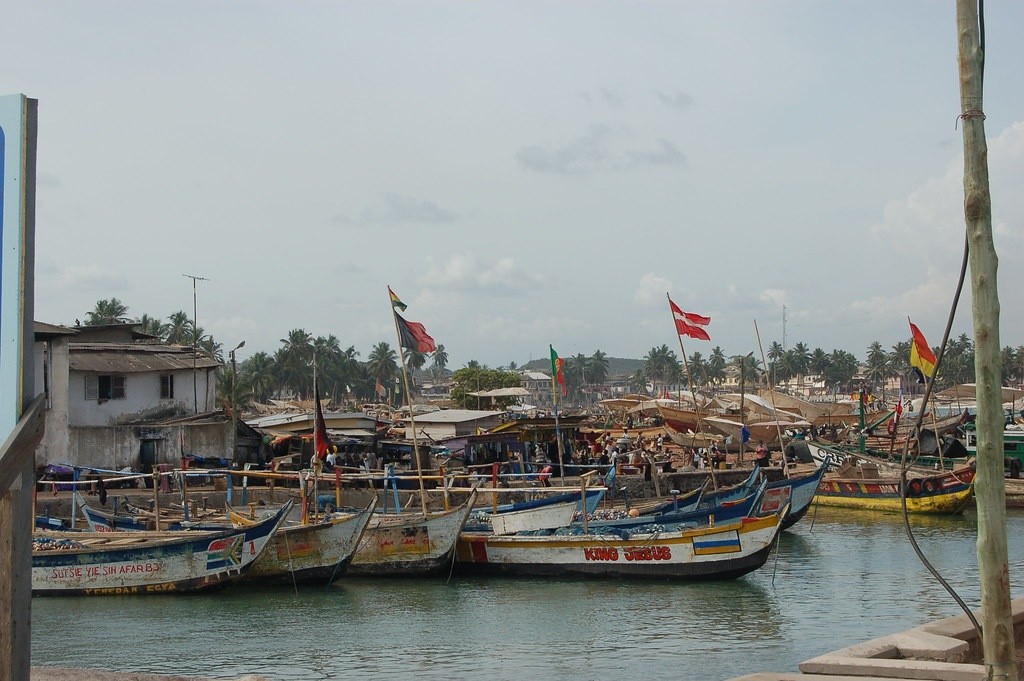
top-left (909, 324), bottom-right (939, 383)
top-left (551, 349), bottom-right (565, 385)
top-left (394, 310), bottom-right (437, 352)
top-left (866, 393), bottom-right (876, 402)
top-left (389, 288), bottom-right (407, 311)
top-left (670, 299), bottom-right (711, 341)
top-left (312, 383), bottom-right (330, 463)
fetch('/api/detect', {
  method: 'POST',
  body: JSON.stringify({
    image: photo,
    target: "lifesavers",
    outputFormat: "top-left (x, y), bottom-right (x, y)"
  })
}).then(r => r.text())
top-left (906, 478), bottom-right (923, 496)
top-left (886, 418), bottom-right (894, 435)
top-left (921, 478), bottom-right (938, 495)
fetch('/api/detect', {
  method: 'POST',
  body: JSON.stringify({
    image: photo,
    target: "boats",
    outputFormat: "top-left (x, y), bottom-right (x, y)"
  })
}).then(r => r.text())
top-left (85, 285), bottom-right (1024, 581)
top-left (31, 492), bottom-right (294, 598)
top-left (33, 468), bottom-right (379, 589)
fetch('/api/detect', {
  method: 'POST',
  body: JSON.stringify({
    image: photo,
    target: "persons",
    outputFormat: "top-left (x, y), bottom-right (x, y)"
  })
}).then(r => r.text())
top-left (627, 416), bottom-right (633, 428)
top-left (692, 446), bottom-right (719, 471)
top-left (787, 420), bottom-right (841, 439)
top-left (755, 440), bottom-right (769, 467)
top-left (622, 430), bottom-right (630, 438)
top-left (311, 445), bottom-right (407, 473)
top-left (1004, 417), bottom-right (1012, 430)
top-left (637, 431), bottom-right (646, 448)
top-left (593, 431), bottom-right (663, 474)
top-left (862, 400), bottom-right (887, 412)
top-left (663, 447), bottom-right (670, 459)
top-left (656, 434), bottom-right (663, 450)
top-left (539, 466), bottom-right (554, 486)
